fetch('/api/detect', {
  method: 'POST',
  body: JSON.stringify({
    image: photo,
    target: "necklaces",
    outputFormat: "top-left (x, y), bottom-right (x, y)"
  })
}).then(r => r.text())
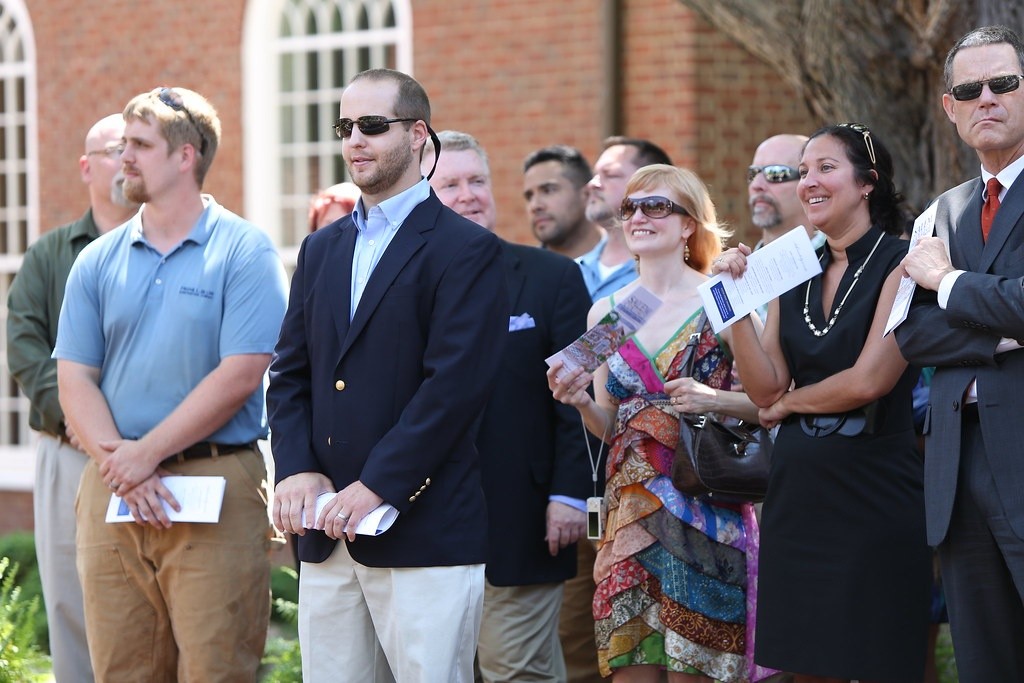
top-left (803, 231), bottom-right (887, 336)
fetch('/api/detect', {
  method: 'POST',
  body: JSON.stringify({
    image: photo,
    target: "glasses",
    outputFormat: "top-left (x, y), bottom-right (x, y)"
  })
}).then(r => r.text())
top-left (747, 165), bottom-right (800, 183)
top-left (149, 87), bottom-right (207, 156)
top-left (621, 196), bottom-right (688, 220)
top-left (837, 121), bottom-right (877, 171)
top-left (332, 116), bottom-right (418, 138)
top-left (89, 144), bottom-right (124, 155)
top-left (950, 75), bottom-right (1024, 101)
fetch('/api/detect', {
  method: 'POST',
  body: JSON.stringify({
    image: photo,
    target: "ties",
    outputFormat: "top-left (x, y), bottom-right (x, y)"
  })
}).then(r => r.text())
top-left (982, 178), bottom-right (1000, 243)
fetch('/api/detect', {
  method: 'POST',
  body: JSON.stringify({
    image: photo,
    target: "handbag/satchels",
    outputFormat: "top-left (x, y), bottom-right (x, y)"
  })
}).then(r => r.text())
top-left (672, 412), bottom-right (773, 501)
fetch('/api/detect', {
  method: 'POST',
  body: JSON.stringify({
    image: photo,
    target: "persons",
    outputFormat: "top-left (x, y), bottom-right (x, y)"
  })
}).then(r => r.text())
top-left (523, 120), bottom-right (934, 683)
top-left (894, 27), bottom-right (1024, 683)
top-left (308, 181), bottom-right (361, 234)
top-left (266, 70), bottom-right (510, 683)
top-left (51, 88), bottom-right (290, 683)
top-left (417, 129), bottom-right (601, 683)
top-left (6, 112), bottom-right (140, 683)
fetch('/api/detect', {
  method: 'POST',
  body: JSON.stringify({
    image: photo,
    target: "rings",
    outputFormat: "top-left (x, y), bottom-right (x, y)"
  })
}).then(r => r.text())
top-left (110, 481), bottom-right (119, 489)
top-left (719, 258), bottom-right (724, 262)
top-left (671, 397), bottom-right (678, 404)
top-left (567, 388), bottom-right (575, 395)
top-left (337, 513), bottom-right (347, 521)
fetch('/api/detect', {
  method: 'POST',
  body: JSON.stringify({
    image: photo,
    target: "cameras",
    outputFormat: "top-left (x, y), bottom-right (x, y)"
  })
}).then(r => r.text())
top-left (587, 497), bottom-right (608, 540)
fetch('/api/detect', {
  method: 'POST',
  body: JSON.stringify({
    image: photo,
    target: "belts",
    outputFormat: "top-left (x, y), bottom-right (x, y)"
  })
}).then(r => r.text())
top-left (159, 443), bottom-right (254, 461)
